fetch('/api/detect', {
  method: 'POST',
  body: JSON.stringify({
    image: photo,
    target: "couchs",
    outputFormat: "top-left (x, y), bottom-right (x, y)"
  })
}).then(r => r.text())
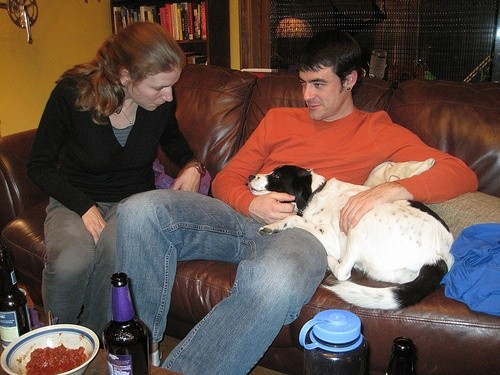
top-left (1, 66), bottom-right (500, 375)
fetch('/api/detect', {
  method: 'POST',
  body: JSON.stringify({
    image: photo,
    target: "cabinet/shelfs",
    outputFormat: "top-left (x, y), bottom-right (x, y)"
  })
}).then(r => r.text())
top-left (110, 0), bottom-right (231, 69)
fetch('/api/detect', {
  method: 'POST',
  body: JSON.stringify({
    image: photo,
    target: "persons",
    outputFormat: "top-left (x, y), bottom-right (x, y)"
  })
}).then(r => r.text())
top-left (22, 23), bottom-right (203, 349)
top-left (108, 30), bottom-right (479, 374)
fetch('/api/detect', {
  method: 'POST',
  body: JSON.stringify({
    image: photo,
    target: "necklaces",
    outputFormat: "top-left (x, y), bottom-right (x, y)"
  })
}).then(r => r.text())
top-left (122, 110), bottom-right (135, 124)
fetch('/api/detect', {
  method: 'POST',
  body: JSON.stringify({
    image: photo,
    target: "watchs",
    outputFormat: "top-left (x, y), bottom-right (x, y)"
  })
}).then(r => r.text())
top-left (179, 162), bottom-right (206, 179)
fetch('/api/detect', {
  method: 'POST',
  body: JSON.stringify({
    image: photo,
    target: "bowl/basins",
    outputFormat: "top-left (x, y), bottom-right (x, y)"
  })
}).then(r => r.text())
top-left (0, 323), bottom-right (100, 375)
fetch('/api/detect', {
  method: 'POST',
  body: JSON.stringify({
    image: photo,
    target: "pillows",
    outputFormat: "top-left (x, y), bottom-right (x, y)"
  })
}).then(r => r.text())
top-left (424, 192), bottom-right (500, 240)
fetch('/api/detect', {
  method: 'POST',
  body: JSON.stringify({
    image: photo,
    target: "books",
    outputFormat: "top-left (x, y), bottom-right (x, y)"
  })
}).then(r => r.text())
top-left (112, 0), bottom-right (207, 41)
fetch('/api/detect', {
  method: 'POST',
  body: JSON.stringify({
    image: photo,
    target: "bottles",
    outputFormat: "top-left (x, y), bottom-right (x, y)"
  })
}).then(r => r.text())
top-left (105, 272), bottom-right (152, 375)
top-left (298, 308), bottom-right (370, 375)
top-left (1, 245), bottom-right (31, 349)
top-left (387, 337), bottom-right (416, 375)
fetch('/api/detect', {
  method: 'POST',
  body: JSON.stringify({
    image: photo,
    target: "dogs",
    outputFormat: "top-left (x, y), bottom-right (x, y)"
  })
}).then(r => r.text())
top-left (249, 164), bottom-right (454, 310)
